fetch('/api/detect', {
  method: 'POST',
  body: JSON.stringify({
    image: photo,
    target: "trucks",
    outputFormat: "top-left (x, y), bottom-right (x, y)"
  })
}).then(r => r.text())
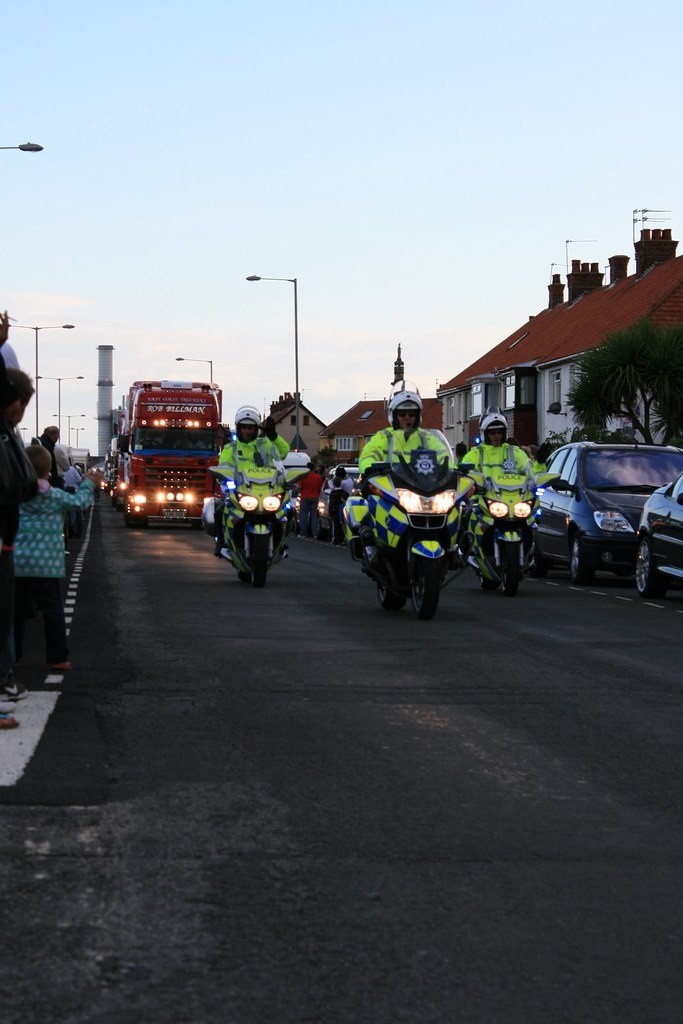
top-left (103, 381), bottom-right (223, 528)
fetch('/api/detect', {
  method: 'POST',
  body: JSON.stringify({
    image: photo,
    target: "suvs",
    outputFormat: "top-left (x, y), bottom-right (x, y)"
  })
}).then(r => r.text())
top-left (527, 441), bottom-right (683, 587)
top-left (317, 463), bottom-right (360, 544)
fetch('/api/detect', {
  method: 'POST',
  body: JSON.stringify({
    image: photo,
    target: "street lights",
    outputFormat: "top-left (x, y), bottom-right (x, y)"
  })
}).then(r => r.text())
top-left (10, 325), bottom-right (75, 440)
top-left (176, 358), bottom-right (212, 384)
top-left (54, 415), bottom-right (86, 446)
top-left (246, 276), bottom-right (308, 451)
top-left (35, 377), bottom-right (84, 442)
top-left (71, 428), bottom-right (85, 447)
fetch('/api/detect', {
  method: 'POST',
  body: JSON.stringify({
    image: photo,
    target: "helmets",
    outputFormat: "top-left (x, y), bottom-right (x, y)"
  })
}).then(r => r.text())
top-left (387, 389), bottom-right (424, 431)
top-left (479, 412), bottom-right (510, 445)
top-left (234, 405), bottom-right (261, 443)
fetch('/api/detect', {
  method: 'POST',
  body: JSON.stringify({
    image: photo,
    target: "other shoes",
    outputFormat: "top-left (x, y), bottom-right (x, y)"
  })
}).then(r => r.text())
top-left (0, 717), bottom-right (19, 730)
top-left (0, 701), bottom-right (18, 714)
top-left (47, 660), bottom-right (72, 671)
top-left (214, 535), bottom-right (224, 557)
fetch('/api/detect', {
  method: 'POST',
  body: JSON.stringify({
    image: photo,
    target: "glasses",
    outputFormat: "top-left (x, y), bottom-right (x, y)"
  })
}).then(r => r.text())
top-left (488, 428), bottom-right (504, 435)
top-left (398, 409), bottom-right (417, 417)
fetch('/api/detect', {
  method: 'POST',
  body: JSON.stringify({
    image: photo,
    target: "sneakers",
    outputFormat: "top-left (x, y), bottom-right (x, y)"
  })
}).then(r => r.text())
top-left (0, 680), bottom-right (29, 701)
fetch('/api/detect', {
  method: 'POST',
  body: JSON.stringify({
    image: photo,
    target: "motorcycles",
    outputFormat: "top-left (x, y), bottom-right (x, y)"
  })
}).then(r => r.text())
top-left (208, 437), bottom-right (310, 588)
top-left (455, 441), bottom-right (561, 597)
top-left (342, 428), bottom-right (475, 621)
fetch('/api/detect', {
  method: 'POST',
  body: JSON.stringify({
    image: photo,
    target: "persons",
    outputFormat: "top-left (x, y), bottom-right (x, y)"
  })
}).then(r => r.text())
top-left (212, 407), bottom-right (292, 559)
top-left (296, 462), bottom-right (323, 541)
top-left (326, 466), bottom-right (354, 546)
top-left (358, 391), bottom-right (551, 561)
top-left (0, 312), bottom-right (103, 730)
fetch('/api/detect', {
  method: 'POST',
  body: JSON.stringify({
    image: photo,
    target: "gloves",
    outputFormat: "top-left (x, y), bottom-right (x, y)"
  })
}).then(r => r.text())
top-left (258, 416), bottom-right (279, 441)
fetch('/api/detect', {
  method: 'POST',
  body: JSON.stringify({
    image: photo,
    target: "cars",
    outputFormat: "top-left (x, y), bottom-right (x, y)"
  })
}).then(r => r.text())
top-left (632, 473), bottom-right (683, 599)
